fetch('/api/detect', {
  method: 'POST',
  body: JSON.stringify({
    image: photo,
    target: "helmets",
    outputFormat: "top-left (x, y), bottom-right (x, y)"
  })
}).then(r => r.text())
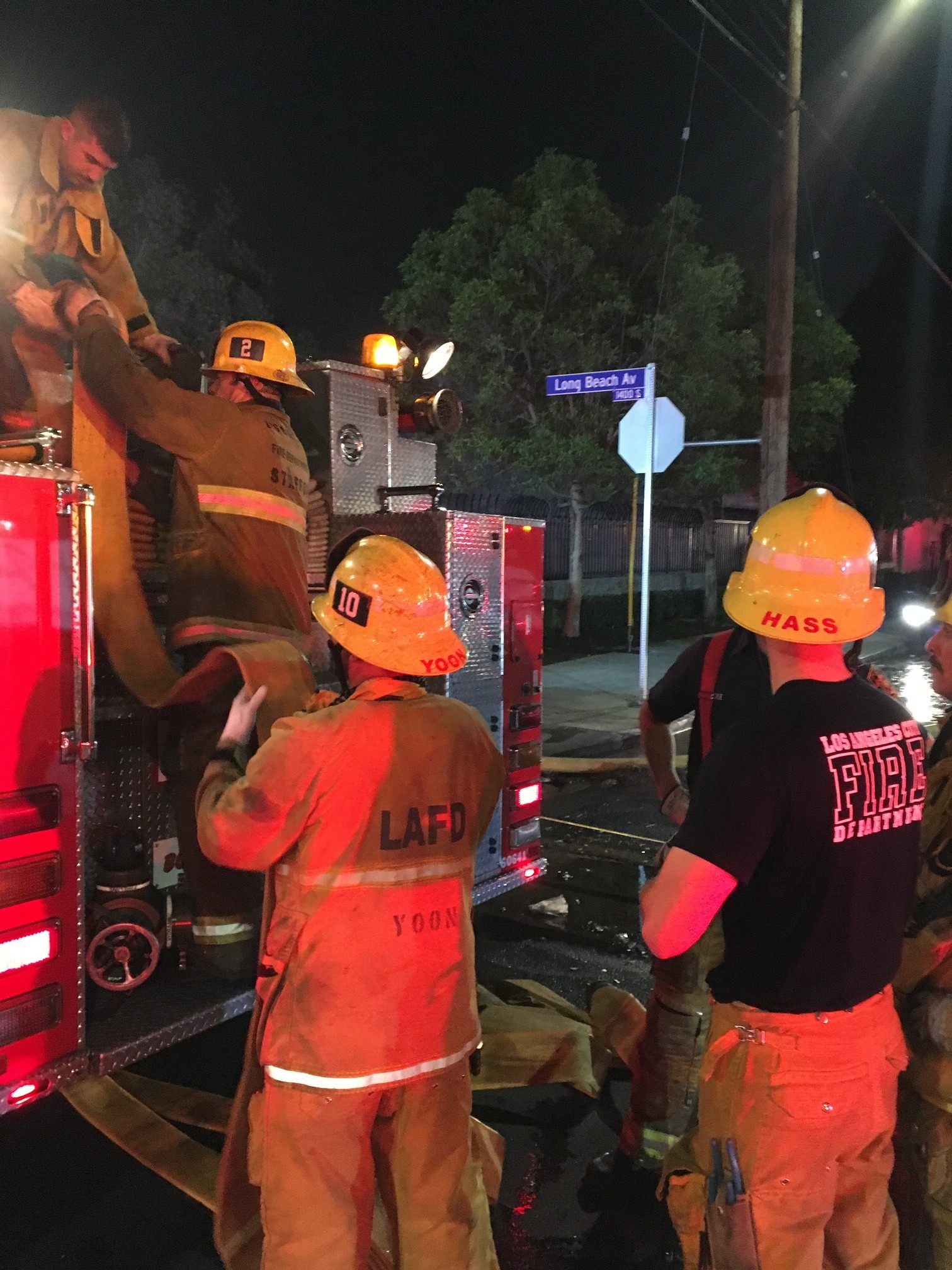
top-left (934, 597), bottom-right (952, 623)
top-left (310, 535), bottom-right (469, 676)
top-left (199, 322), bottom-right (314, 393)
top-left (720, 488), bottom-right (886, 643)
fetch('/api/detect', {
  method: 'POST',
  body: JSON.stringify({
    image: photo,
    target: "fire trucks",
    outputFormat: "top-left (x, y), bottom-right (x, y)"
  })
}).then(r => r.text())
top-left (1, 332), bottom-right (553, 1122)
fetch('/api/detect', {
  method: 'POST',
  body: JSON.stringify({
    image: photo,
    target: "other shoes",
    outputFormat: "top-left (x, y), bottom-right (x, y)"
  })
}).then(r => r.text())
top-left (194, 942), bottom-right (243, 969)
top-left (592, 1148), bottom-right (641, 1175)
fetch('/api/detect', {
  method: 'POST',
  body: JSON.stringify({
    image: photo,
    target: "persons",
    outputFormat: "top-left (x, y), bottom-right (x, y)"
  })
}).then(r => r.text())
top-left (195, 527), bottom-right (507, 1270)
top-left (57, 280), bottom-right (313, 981)
top-left (1, 79), bottom-right (186, 471)
top-left (635, 485), bottom-right (952, 1268)
